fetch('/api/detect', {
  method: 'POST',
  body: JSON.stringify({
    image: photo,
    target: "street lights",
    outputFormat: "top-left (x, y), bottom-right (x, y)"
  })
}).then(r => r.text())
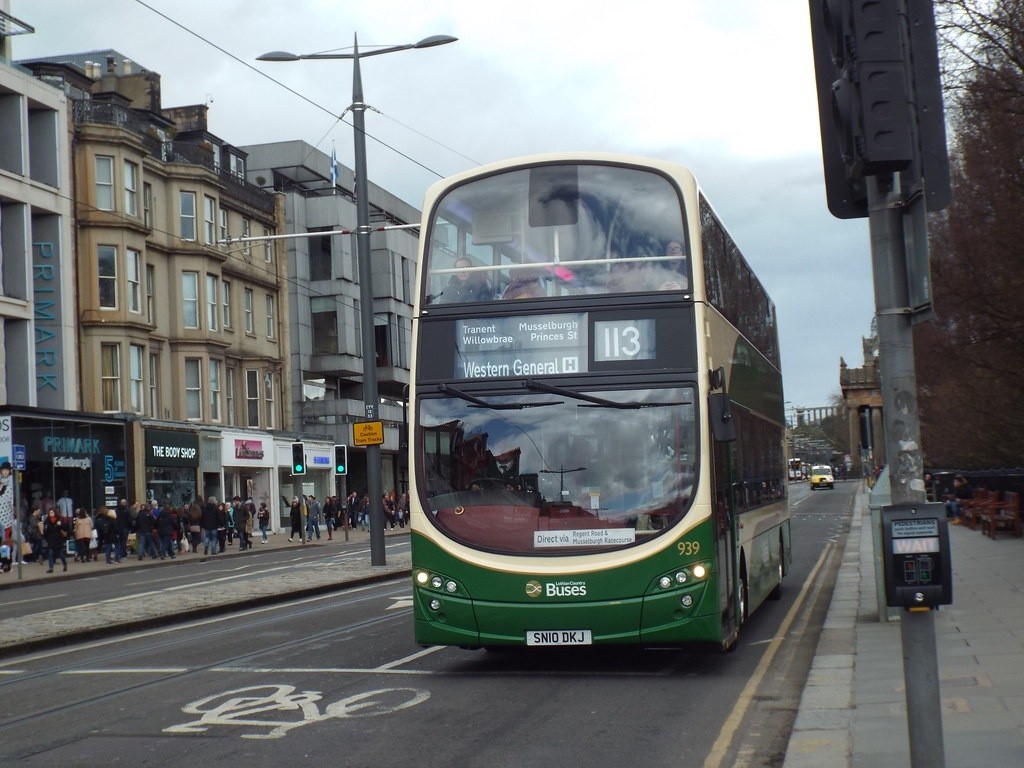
top-left (255, 33), bottom-right (458, 568)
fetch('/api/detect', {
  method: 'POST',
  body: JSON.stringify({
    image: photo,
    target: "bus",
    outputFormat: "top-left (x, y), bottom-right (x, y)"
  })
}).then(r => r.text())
top-left (788, 458), bottom-right (802, 480)
top-left (801, 462), bottom-right (811, 479)
top-left (407, 151), bottom-right (791, 657)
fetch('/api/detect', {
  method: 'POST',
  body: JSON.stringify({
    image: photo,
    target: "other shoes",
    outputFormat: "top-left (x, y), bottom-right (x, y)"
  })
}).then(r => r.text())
top-left (288, 538), bottom-right (293, 542)
top-left (297, 538), bottom-right (302, 542)
top-left (262, 540), bottom-right (268, 544)
top-left (63, 564), bottom-right (68, 571)
top-left (0, 569), bottom-right (4, 574)
top-left (73, 554), bottom-right (176, 564)
top-left (239, 546), bottom-right (246, 551)
top-left (46, 569), bottom-right (53, 572)
top-left (307, 539), bottom-right (311, 542)
top-left (249, 542), bottom-right (252, 548)
top-left (192, 542), bottom-right (233, 555)
top-left (391, 528), bottom-right (393, 530)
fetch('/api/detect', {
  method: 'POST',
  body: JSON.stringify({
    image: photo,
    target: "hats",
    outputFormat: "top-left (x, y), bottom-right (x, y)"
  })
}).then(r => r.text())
top-left (0, 462), bottom-right (12, 470)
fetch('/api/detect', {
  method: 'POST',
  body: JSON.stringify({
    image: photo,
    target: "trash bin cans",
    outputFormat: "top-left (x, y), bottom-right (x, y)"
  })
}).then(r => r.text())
top-left (869, 464), bottom-right (946, 623)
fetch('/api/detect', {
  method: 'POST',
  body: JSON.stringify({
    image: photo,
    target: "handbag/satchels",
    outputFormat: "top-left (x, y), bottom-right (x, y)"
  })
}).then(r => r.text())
top-left (21, 542), bottom-right (32, 555)
top-left (89, 533), bottom-right (98, 549)
top-left (38, 523), bottom-right (44, 535)
top-left (190, 525), bottom-right (201, 532)
top-left (60, 528), bottom-right (67, 537)
top-left (245, 511), bottom-right (252, 534)
top-left (398, 511), bottom-right (403, 519)
top-left (181, 534), bottom-right (189, 552)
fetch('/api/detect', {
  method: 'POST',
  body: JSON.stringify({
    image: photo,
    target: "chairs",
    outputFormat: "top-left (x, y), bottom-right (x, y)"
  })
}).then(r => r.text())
top-left (500, 265), bottom-right (548, 301)
top-left (603, 259), bottom-right (650, 294)
top-left (942, 487), bottom-right (1021, 540)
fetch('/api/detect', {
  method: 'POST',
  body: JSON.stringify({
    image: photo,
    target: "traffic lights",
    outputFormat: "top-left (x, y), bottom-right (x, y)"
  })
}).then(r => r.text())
top-left (334, 445), bottom-right (347, 475)
top-left (291, 442), bottom-right (306, 475)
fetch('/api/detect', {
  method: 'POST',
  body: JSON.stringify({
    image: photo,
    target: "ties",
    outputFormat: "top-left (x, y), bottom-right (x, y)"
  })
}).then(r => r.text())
top-left (500, 466), bottom-right (507, 474)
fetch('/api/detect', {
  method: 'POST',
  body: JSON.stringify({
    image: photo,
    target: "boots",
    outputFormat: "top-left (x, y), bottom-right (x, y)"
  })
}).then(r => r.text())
top-left (328, 528), bottom-right (332, 540)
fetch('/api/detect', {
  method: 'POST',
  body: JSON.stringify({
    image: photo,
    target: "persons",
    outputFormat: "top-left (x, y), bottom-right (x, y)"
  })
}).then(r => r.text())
top-left (306, 495), bottom-right (343, 542)
top-left (651, 241), bottom-right (687, 291)
top-left (72, 496), bottom-right (256, 564)
top-left (841, 464), bottom-right (847, 480)
top-left (873, 466), bottom-right (880, 480)
top-left (28, 505), bottom-right (68, 573)
top-left (288, 496), bottom-right (302, 542)
top-left (925, 474), bottom-right (932, 492)
top-left (440, 257), bottom-right (490, 303)
top-left (0, 513), bottom-right (27, 573)
top-left (338, 492), bottom-right (370, 532)
top-left (257, 503), bottom-right (269, 544)
top-left (469, 436), bottom-right (527, 492)
top-left (57, 490), bottom-right (73, 532)
top-left (503, 268), bottom-right (545, 299)
top-left (0, 462), bottom-right (14, 545)
top-left (944, 474), bottom-right (972, 524)
top-left (383, 489), bottom-right (410, 530)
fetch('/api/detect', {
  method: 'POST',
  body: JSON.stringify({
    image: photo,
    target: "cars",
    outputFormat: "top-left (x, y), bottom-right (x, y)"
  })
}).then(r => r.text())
top-left (810, 465), bottom-right (835, 490)
